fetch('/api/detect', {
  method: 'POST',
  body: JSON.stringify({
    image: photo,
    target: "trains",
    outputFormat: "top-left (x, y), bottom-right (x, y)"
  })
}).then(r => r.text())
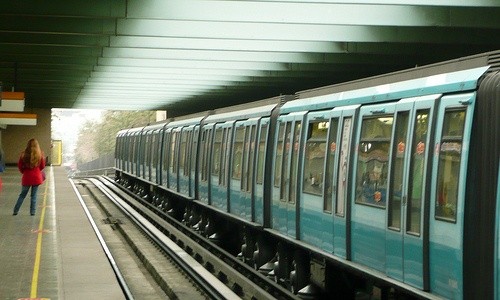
top-left (114, 49), bottom-right (499, 299)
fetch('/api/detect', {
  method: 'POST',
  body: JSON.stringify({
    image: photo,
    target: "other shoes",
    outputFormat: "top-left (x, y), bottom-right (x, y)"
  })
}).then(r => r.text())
top-left (13, 212), bottom-right (17, 215)
top-left (31, 213), bottom-right (34, 216)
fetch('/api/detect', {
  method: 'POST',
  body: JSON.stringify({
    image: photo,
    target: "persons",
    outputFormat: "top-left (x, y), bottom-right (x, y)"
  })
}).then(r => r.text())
top-left (13, 138), bottom-right (46, 216)
